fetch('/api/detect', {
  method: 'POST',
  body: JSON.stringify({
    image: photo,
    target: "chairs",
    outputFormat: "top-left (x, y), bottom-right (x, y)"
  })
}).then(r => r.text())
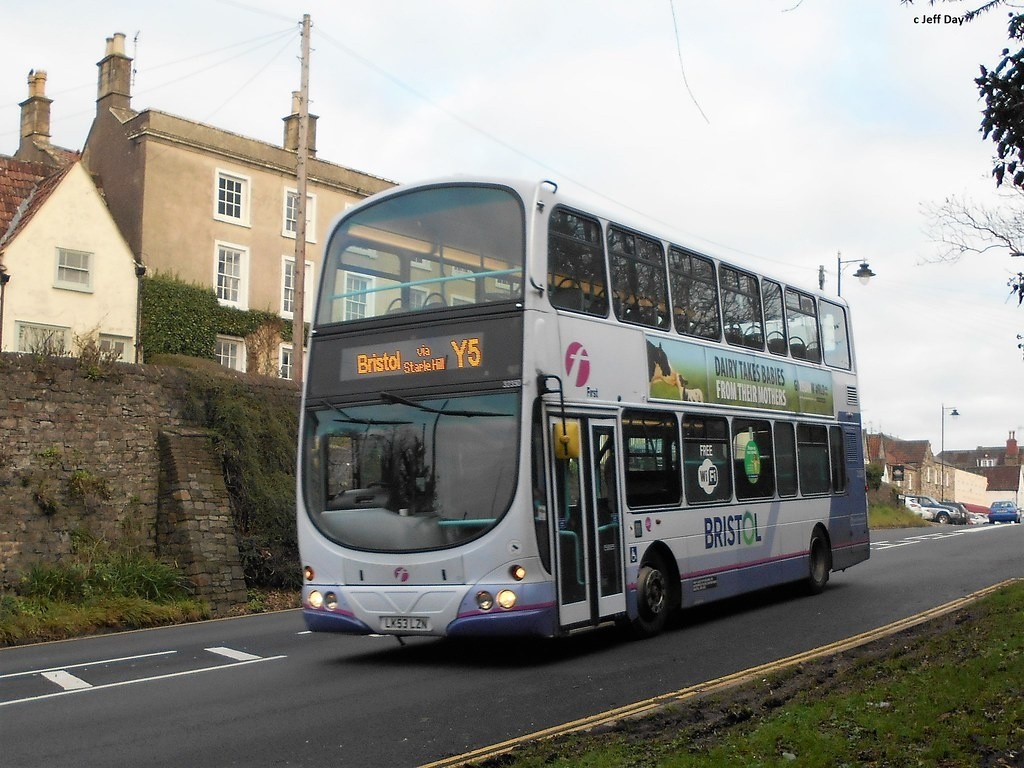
top-left (383, 275), bottom-right (825, 368)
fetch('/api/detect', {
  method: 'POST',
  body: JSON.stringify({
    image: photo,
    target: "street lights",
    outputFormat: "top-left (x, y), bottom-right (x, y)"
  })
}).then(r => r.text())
top-left (836, 251), bottom-right (876, 294)
top-left (941, 403), bottom-right (959, 500)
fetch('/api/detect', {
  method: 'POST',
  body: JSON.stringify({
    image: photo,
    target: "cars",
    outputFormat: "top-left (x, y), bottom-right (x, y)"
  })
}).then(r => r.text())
top-left (898, 496), bottom-right (989, 527)
top-left (987, 501), bottom-right (1021, 524)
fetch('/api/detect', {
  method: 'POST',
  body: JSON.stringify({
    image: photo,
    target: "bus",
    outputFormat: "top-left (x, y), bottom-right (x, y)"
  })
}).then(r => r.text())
top-left (297, 172), bottom-right (872, 643)
